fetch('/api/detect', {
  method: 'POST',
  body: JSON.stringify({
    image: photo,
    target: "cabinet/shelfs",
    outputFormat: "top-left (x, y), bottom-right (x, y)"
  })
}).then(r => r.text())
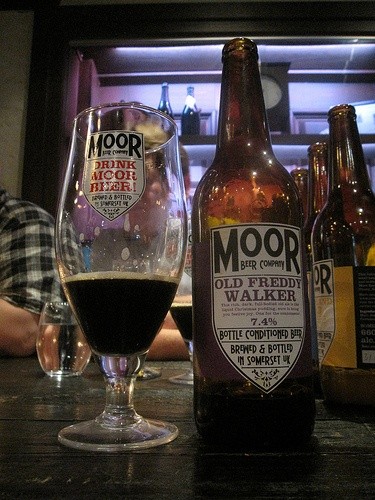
top-left (73, 50), bottom-right (375, 222)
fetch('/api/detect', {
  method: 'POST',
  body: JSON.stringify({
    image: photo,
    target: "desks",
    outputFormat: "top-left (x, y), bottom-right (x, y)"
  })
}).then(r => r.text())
top-left (0, 349), bottom-right (375, 500)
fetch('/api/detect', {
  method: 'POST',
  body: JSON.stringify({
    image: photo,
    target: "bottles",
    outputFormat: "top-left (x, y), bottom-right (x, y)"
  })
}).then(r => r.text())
top-left (158, 82), bottom-right (174, 120)
top-left (181, 86), bottom-right (200, 135)
top-left (192, 39), bottom-right (319, 447)
top-left (291, 104), bottom-right (375, 414)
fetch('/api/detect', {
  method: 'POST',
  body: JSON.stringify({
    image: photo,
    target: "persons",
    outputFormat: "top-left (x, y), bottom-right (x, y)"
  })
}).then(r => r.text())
top-left (0, 188), bottom-right (66, 356)
top-left (81, 144), bottom-right (191, 359)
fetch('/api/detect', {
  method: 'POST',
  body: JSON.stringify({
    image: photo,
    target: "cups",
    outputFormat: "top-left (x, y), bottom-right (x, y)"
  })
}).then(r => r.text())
top-left (35, 302), bottom-right (90, 376)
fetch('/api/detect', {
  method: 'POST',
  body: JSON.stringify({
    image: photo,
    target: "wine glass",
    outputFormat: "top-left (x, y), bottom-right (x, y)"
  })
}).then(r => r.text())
top-left (53, 102), bottom-right (187, 453)
top-left (169, 164), bottom-right (194, 387)
